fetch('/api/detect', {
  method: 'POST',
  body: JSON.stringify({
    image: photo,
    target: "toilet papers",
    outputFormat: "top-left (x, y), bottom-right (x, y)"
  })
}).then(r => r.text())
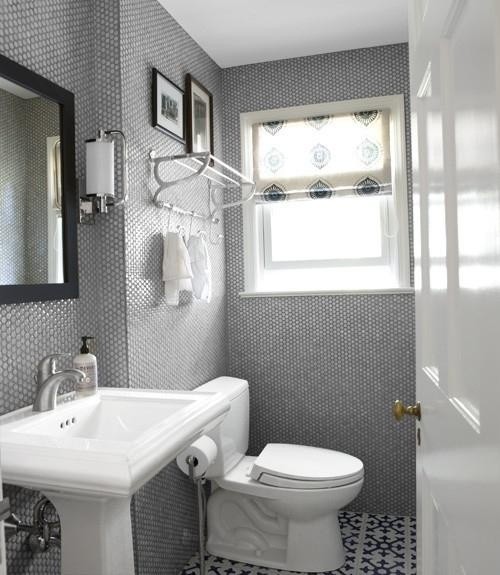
top-left (175, 434), bottom-right (219, 478)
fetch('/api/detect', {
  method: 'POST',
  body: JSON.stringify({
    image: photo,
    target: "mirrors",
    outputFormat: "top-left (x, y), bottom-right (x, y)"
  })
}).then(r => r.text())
top-left (1, 53), bottom-right (80, 304)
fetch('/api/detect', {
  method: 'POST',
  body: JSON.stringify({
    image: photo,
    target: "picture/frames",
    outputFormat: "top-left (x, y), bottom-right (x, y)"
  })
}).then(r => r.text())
top-left (150, 67), bottom-right (215, 168)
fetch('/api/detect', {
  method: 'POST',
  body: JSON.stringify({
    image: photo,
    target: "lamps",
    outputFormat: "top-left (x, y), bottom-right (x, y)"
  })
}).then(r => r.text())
top-left (79, 127), bottom-right (127, 224)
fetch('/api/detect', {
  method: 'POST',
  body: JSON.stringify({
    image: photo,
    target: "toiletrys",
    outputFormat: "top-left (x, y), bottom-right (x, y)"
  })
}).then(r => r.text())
top-left (72, 335), bottom-right (99, 397)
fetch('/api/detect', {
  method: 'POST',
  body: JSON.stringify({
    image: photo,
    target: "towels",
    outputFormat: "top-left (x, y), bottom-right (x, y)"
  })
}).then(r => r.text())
top-left (161, 230), bottom-right (213, 307)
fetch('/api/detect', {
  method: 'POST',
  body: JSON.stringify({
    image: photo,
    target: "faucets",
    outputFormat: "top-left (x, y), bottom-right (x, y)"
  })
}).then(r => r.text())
top-left (32, 352), bottom-right (90, 411)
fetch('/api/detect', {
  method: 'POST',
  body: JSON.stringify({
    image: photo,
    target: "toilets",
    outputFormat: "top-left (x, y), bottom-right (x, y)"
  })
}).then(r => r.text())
top-left (191, 374), bottom-right (366, 573)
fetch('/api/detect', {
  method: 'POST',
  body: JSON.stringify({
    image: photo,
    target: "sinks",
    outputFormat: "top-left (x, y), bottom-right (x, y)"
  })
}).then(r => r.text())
top-left (0, 387), bottom-right (231, 497)
